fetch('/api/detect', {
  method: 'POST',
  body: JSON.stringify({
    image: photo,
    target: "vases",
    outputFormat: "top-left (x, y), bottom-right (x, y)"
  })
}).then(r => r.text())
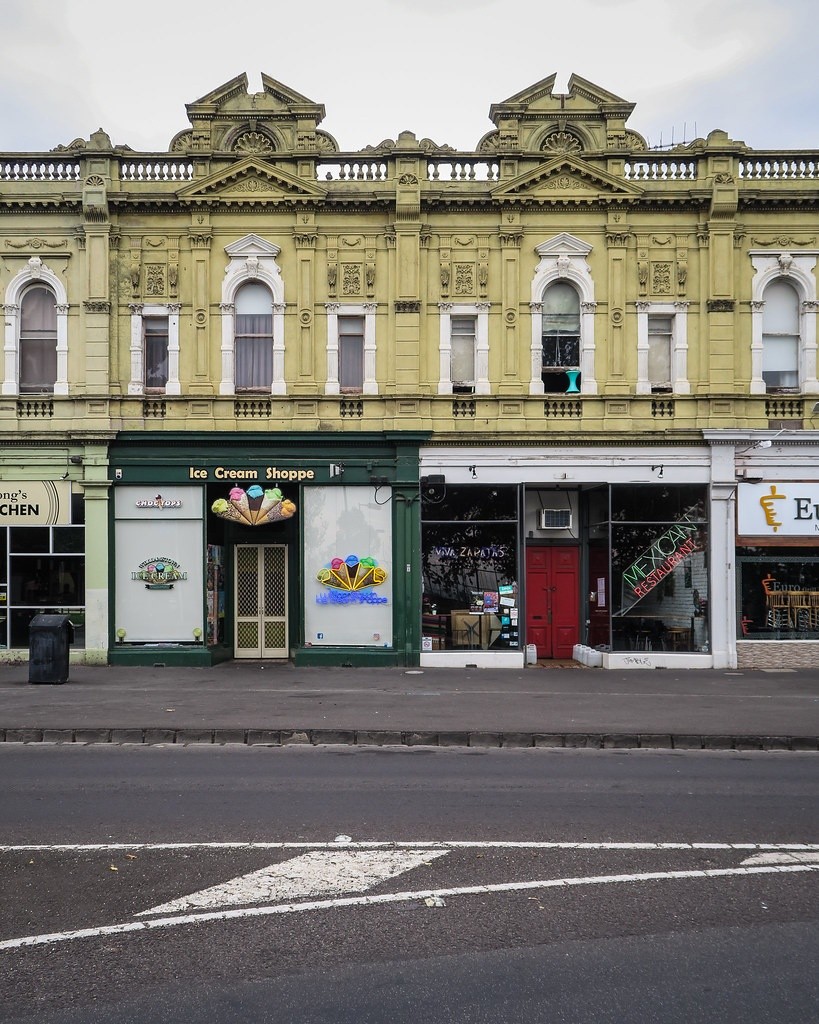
top-left (565, 371), bottom-right (581, 393)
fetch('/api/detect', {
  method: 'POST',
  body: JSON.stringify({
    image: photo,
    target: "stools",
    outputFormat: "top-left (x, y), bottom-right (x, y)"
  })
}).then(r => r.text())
top-left (635, 627), bottom-right (695, 651)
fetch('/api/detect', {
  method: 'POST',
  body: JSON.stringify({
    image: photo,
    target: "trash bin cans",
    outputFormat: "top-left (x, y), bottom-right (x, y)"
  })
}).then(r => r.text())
top-left (28, 613), bottom-right (72, 685)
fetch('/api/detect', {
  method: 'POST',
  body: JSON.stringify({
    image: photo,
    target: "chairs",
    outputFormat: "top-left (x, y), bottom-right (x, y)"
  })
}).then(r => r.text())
top-left (763, 589), bottom-right (819, 630)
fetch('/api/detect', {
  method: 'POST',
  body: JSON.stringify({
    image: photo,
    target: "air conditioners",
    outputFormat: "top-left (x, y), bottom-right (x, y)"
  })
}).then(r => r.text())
top-left (71, 455), bottom-right (83, 463)
top-left (537, 507), bottom-right (573, 530)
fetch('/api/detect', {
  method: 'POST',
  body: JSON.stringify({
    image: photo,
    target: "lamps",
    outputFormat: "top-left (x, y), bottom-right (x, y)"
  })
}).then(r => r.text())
top-left (60, 471), bottom-right (70, 480)
top-left (652, 464), bottom-right (665, 478)
top-left (330, 463), bottom-right (344, 478)
top-left (468, 464), bottom-right (478, 480)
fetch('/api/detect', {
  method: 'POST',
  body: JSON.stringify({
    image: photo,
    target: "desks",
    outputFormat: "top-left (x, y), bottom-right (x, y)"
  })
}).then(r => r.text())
top-left (456, 612), bottom-right (505, 650)
